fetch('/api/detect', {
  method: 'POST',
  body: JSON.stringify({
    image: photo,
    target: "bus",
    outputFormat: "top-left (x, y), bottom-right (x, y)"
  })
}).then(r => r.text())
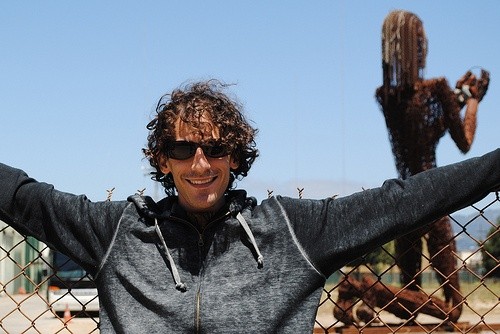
top-left (45, 246), bottom-right (99, 316)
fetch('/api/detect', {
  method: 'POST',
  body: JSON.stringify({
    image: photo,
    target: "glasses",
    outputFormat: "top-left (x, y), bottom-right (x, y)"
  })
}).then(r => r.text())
top-left (157, 138), bottom-right (238, 160)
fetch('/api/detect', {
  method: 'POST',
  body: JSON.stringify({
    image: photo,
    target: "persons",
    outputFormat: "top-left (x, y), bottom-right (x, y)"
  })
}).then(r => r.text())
top-left (333, 10), bottom-right (490, 324)
top-left (0, 77), bottom-right (500, 334)
